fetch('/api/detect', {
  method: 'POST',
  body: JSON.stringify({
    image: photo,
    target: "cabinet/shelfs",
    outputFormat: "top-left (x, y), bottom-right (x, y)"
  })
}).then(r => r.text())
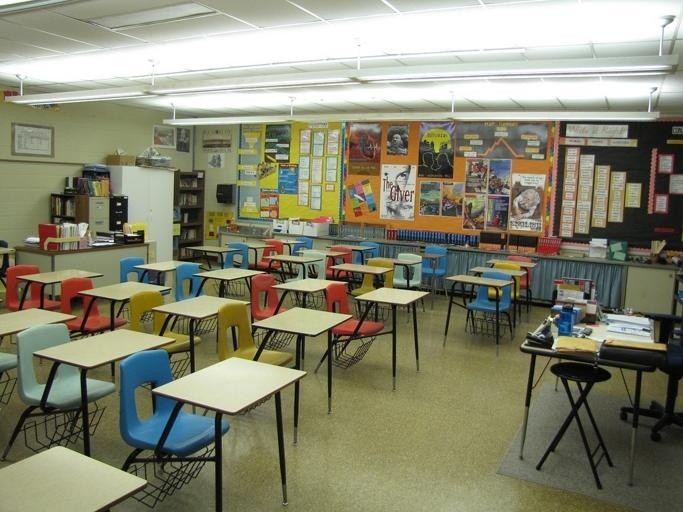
top-left (75, 194), bottom-right (110, 240)
top-left (106, 165), bottom-right (174, 263)
top-left (51, 192), bottom-right (76, 224)
top-left (172, 168), bottom-right (206, 262)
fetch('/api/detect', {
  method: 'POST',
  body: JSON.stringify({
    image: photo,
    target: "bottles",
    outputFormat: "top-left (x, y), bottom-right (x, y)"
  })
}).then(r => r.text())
top-left (586, 300), bottom-right (596, 324)
top-left (558, 304), bottom-right (574, 336)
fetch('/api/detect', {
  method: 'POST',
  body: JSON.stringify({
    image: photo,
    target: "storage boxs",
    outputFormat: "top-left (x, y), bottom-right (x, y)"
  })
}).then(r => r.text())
top-left (136, 155), bottom-right (172, 167)
top-left (107, 154), bottom-right (136, 166)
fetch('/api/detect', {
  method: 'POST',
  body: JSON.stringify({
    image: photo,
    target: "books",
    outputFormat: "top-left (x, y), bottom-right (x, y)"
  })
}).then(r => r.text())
top-left (22, 169), bottom-right (148, 252)
top-left (172, 176), bottom-right (201, 258)
top-left (546, 276), bottom-right (671, 369)
top-left (588, 237), bottom-right (630, 262)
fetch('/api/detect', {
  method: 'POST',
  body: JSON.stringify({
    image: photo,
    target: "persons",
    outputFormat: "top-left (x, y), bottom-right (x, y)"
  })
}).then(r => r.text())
top-left (154, 125), bottom-right (175, 146)
top-left (382, 125), bottom-right (540, 229)
top-left (178, 128), bottom-right (190, 152)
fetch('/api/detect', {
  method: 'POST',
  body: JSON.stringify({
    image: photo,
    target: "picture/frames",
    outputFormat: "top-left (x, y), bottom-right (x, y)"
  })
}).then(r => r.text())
top-left (153, 125), bottom-right (177, 149)
top-left (11, 122), bottom-right (55, 158)
